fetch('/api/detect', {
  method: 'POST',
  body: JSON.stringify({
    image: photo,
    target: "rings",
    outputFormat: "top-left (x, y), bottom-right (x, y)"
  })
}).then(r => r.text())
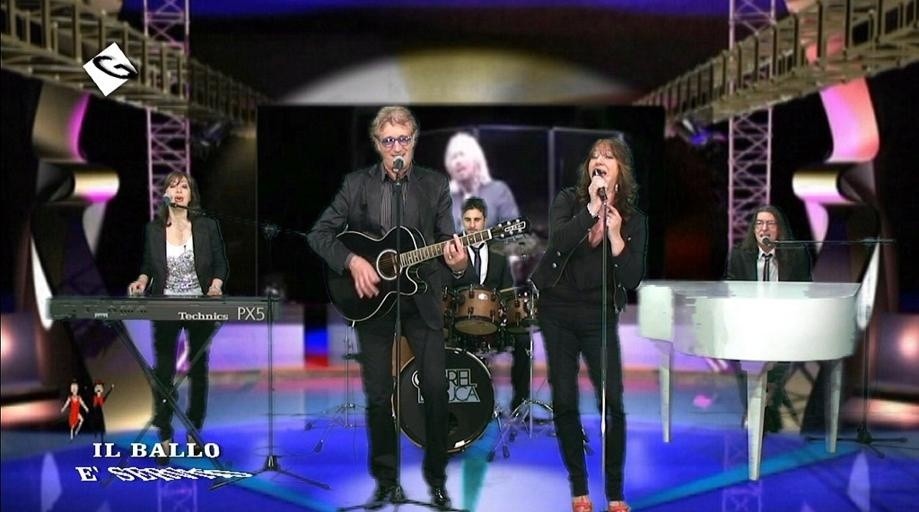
top-left (448, 255), bottom-right (452, 259)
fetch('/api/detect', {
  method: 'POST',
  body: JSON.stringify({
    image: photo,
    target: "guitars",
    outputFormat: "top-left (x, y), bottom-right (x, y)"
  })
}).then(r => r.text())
top-left (325, 216), bottom-right (533, 326)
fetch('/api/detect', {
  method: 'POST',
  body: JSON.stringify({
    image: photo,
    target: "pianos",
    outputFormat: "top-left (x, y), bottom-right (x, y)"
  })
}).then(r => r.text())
top-left (50, 295), bottom-right (282, 325)
top-left (637, 280), bottom-right (860, 481)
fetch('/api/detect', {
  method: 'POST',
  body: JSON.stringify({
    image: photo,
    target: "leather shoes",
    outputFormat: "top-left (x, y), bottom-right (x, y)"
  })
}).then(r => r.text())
top-left (365, 482), bottom-right (398, 512)
top-left (427, 482), bottom-right (451, 510)
top-left (186, 432), bottom-right (204, 457)
top-left (607, 500), bottom-right (629, 512)
top-left (156, 436), bottom-right (173, 466)
top-left (571, 496), bottom-right (592, 512)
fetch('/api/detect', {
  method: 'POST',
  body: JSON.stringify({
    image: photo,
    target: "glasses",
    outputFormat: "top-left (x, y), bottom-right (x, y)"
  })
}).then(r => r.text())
top-left (375, 133), bottom-right (411, 148)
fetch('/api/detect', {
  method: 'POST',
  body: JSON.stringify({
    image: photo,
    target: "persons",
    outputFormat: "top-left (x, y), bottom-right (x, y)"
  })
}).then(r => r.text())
top-left (725, 199), bottom-right (807, 433)
top-left (128, 166), bottom-right (229, 454)
top-left (533, 133), bottom-right (651, 511)
top-left (443, 133), bottom-right (524, 284)
top-left (308, 105), bottom-right (480, 511)
top-left (457, 198), bottom-right (533, 420)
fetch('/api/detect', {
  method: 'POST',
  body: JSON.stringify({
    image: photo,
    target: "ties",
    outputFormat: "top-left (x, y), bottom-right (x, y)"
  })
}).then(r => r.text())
top-left (470, 242), bottom-right (485, 284)
top-left (762, 253), bottom-right (773, 281)
top-left (390, 178), bottom-right (405, 227)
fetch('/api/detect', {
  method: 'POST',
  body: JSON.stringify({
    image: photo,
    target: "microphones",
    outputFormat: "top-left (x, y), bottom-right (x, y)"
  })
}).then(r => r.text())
top-left (761, 235), bottom-right (774, 249)
top-left (392, 156), bottom-right (405, 172)
top-left (162, 192), bottom-right (172, 206)
top-left (592, 168), bottom-right (607, 201)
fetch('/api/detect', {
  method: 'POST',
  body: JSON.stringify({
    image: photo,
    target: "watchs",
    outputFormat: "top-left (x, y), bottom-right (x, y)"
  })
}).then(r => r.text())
top-left (449, 268), bottom-right (465, 276)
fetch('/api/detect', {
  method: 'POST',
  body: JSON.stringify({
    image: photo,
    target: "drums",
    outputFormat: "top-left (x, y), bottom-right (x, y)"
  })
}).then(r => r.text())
top-left (499, 287), bottom-right (542, 335)
top-left (453, 286), bottom-right (502, 335)
top-left (443, 289), bottom-right (454, 339)
top-left (393, 346), bottom-right (495, 456)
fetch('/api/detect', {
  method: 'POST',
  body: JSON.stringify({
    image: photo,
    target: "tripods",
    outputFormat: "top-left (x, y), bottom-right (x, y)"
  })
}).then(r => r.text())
top-left (805, 237), bottom-right (908, 459)
top-left (304, 323), bottom-right (369, 454)
top-left (208, 216), bottom-right (332, 491)
top-left (480, 321), bottom-right (593, 461)
